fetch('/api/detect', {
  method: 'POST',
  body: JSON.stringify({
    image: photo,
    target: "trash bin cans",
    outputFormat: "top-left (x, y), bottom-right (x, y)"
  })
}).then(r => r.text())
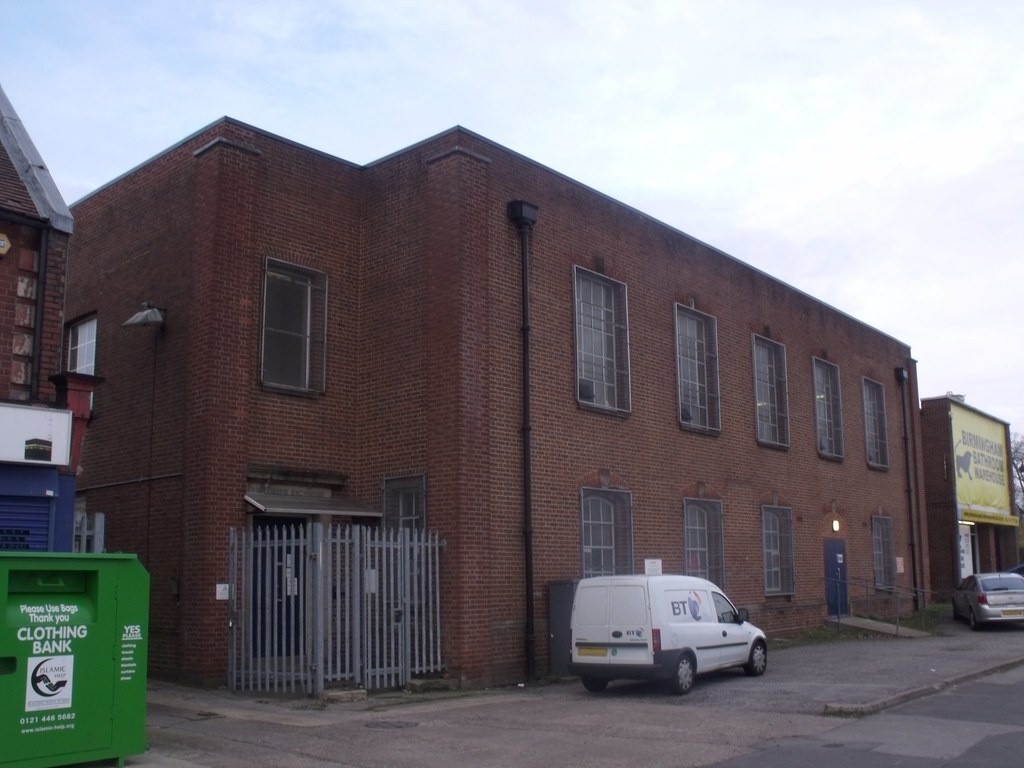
top-left (0, 552), bottom-right (151, 768)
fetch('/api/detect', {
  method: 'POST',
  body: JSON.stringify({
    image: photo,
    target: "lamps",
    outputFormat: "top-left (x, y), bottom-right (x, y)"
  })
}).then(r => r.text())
top-left (122, 302), bottom-right (168, 327)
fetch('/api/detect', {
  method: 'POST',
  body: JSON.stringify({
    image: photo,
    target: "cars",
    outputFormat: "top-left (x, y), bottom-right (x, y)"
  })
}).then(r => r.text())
top-left (949, 563), bottom-right (1024, 631)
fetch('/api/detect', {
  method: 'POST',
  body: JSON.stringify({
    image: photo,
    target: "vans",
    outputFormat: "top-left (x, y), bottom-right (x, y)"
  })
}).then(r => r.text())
top-left (567, 572), bottom-right (768, 695)
